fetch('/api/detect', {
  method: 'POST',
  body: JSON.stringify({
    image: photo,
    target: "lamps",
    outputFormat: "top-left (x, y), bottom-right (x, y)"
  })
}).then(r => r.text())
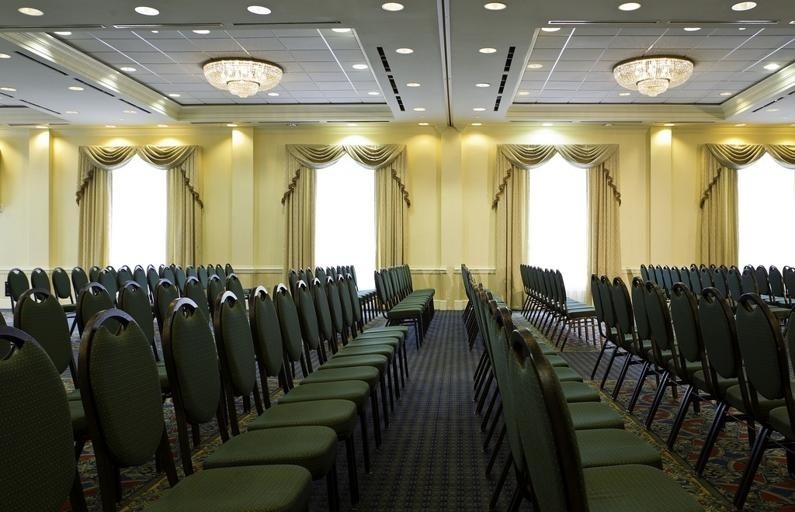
top-left (203, 60), bottom-right (284, 99)
top-left (613, 57), bottom-right (694, 97)
